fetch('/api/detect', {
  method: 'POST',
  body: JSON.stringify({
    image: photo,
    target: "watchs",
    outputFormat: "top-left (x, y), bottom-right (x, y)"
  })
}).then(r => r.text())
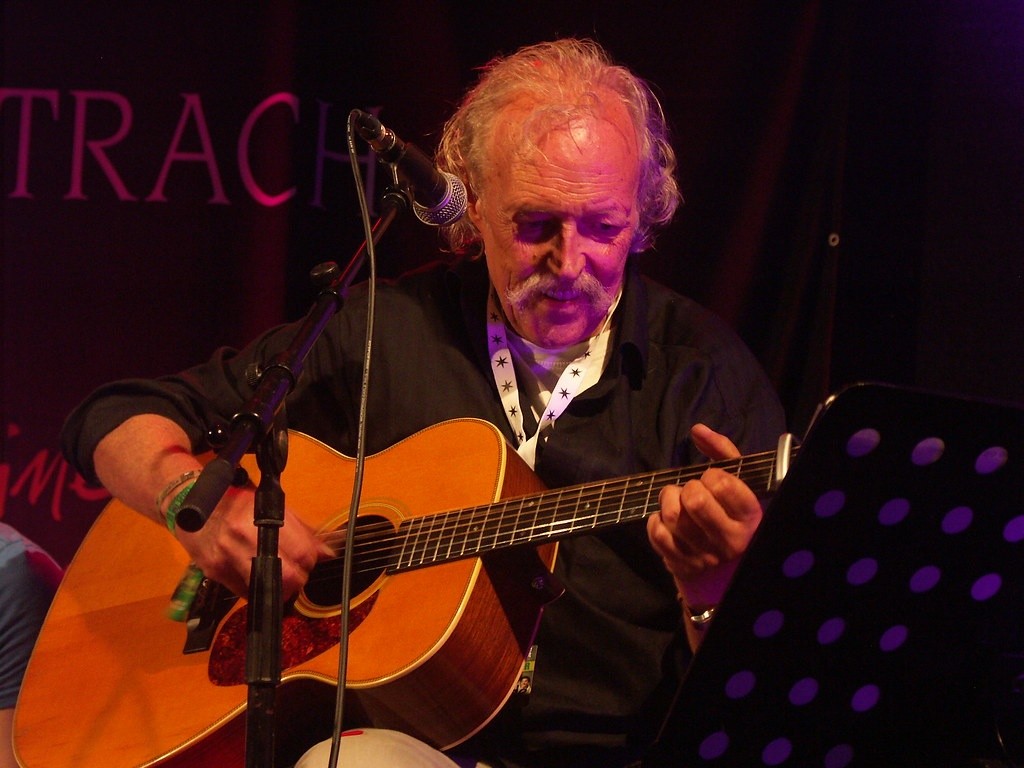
top-left (678, 592), bottom-right (719, 625)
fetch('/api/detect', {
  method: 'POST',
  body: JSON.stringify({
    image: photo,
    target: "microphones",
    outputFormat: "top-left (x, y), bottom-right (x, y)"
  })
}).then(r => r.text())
top-left (350, 107), bottom-right (467, 227)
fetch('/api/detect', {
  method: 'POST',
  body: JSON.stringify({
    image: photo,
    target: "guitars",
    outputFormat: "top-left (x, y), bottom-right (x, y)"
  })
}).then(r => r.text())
top-left (12, 415), bottom-right (802, 768)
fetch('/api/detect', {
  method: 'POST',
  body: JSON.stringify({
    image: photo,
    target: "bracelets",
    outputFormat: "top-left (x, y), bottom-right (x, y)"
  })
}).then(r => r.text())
top-left (167, 478), bottom-right (205, 620)
top-left (156, 469), bottom-right (207, 530)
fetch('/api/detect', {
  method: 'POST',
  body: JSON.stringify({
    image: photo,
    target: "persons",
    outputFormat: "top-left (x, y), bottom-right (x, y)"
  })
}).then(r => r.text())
top-left (58, 34), bottom-right (786, 767)
top-left (0, 520), bottom-right (64, 768)
